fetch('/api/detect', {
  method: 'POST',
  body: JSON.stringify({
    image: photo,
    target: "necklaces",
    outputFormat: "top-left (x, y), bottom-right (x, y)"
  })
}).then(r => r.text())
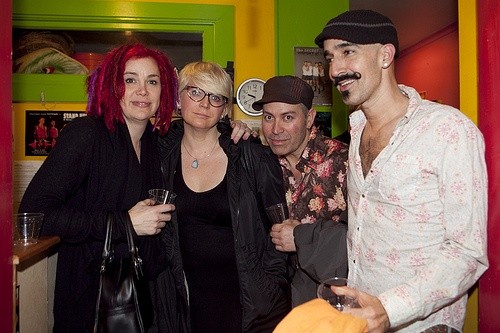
top-left (181, 137), bottom-right (218, 168)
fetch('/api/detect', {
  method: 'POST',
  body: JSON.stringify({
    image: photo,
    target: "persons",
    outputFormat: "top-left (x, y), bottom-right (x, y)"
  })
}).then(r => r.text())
top-left (252, 75), bottom-right (350, 311)
top-left (17, 42), bottom-right (259, 333)
top-left (30, 117), bottom-right (68, 154)
top-left (154, 60), bottom-right (295, 333)
top-left (314, 8), bottom-right (491, 333)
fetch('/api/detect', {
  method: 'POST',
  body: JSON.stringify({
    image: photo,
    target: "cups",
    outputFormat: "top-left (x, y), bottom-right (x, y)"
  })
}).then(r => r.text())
top-left (147, 188), bottom-right (177, 213)
top-left (316, 277), bottom-right (359, 312)
top-left (264, 202), bottom-right (290, 224)
top-left (13, 213), bottom-right (45, 246)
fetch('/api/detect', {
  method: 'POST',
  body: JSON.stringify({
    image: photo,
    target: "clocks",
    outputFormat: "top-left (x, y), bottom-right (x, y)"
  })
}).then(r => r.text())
top-left (235, 78), bottom-right (266, 117)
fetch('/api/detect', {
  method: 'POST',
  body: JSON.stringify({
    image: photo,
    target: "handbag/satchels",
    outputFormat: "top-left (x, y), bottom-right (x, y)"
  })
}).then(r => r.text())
top-left (91, 210), bottom-right (149, 333)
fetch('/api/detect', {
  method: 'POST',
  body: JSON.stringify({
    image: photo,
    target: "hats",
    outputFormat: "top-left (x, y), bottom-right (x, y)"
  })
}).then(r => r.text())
top-left (314, 9), bottom-right (399, 59)
top-left (252, 75), bottom-right (314, 111)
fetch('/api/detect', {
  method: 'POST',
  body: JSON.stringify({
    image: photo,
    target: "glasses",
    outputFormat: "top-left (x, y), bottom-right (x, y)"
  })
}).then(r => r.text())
top-left (185, 85), bottom-right (228, 107)
